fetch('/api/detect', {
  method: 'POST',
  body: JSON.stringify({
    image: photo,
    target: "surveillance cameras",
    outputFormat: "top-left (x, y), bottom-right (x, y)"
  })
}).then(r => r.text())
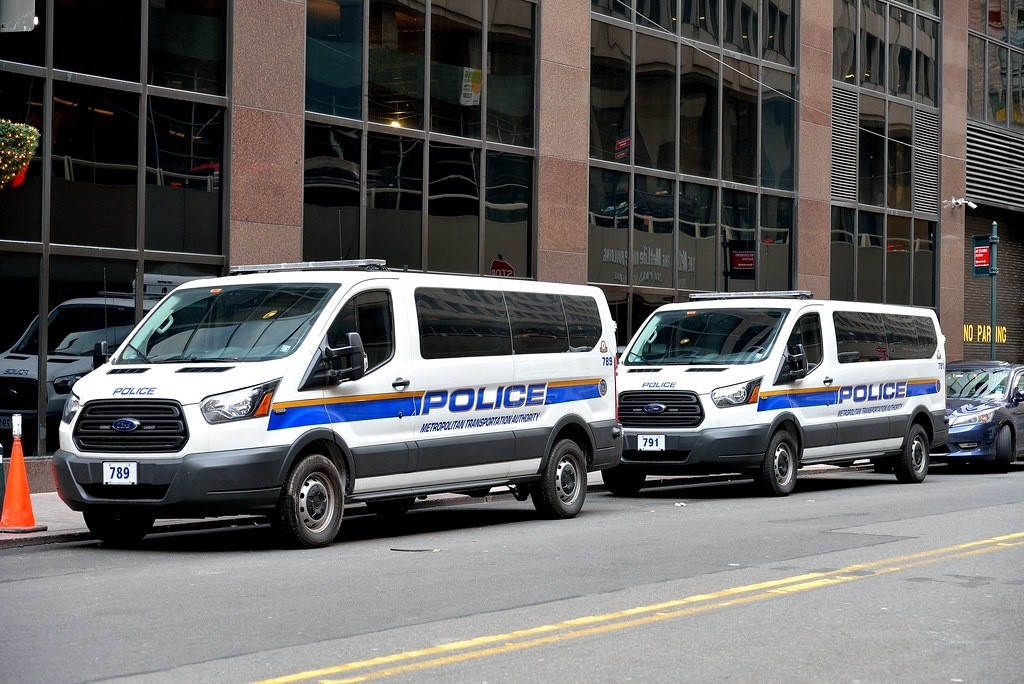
top-left (968, 201), bottom-right (977, 208)
top-left (954, 200), bottom-right (963, 207)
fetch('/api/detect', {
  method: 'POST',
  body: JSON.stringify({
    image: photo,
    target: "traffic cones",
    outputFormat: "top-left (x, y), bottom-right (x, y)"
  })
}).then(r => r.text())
top-left (0, 437), bottom-right (49, 533)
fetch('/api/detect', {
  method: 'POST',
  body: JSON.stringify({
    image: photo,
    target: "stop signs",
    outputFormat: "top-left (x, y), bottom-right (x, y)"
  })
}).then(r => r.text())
top-left (487, 260), bottom-right (515, 278)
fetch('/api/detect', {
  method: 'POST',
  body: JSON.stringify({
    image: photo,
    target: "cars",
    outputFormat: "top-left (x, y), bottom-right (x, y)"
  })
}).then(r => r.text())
top-left (869, 359), bottom-right (1024, 473)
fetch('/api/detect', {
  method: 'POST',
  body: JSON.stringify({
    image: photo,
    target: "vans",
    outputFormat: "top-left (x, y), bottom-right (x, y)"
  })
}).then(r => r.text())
top-left (0, 290), bottom-right (208, 464)
top-left (51, 256), bottom-right (623, 547)
top-left (601, 290), bottom-right (948, 497)
top-left (131, 247), bottom-right (223, 293)
